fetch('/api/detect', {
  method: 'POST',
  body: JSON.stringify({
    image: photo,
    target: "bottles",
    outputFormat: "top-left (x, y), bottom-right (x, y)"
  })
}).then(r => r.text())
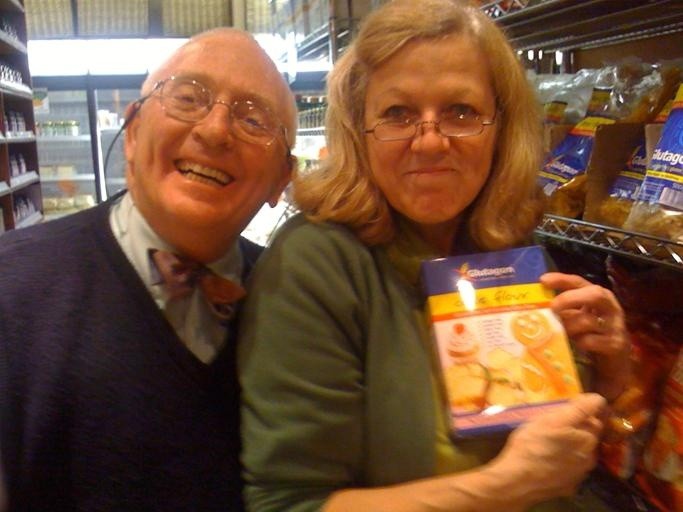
top-left (1, 16), bottom-right (35, 229)
top-left (302, 159), bottom-right (326, 175)
top-left (290, 93), bottom-right (327, 128)
top-left (34, 119), bottom-right (79, 135)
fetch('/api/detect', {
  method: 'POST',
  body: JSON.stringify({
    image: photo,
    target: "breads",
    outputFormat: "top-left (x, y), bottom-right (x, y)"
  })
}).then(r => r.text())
top-left (444, 361), bottom-right (491, 413)
top-left (540, 178), bottom-right (682, 258)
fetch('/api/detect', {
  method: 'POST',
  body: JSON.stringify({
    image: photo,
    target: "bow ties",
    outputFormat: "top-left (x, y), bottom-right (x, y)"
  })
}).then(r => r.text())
top-left (145, 245), bottom-right (246, 322)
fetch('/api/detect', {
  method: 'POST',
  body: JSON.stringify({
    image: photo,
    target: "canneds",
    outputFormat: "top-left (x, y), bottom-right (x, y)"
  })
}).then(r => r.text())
top-left (34, 120), bottom-right (78, 138)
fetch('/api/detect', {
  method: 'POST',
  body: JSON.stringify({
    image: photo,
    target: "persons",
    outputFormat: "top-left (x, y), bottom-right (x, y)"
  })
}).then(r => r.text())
top-left (0, 24), bottom-right (297, 512)
top-left (233, 1), bottom-right (637, 511)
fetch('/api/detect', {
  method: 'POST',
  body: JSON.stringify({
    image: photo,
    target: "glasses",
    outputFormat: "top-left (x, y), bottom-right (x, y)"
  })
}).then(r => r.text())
top-left (145, 76), bottom-right (292, 160)
top-left (363, 99), bottom-right (501, 142)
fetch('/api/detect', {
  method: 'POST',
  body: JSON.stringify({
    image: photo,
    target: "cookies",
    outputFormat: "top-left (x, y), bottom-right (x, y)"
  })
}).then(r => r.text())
top-left (488, 311), bottom-right (578, 407)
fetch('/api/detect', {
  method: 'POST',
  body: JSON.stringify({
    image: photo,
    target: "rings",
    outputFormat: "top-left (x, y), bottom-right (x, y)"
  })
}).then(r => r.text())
top-left (595, 315), bottom-right (605, 331)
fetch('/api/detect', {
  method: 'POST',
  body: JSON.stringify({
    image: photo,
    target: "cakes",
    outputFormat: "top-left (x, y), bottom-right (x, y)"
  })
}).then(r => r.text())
top-left (444, 324), bottom-right (481, 363)
